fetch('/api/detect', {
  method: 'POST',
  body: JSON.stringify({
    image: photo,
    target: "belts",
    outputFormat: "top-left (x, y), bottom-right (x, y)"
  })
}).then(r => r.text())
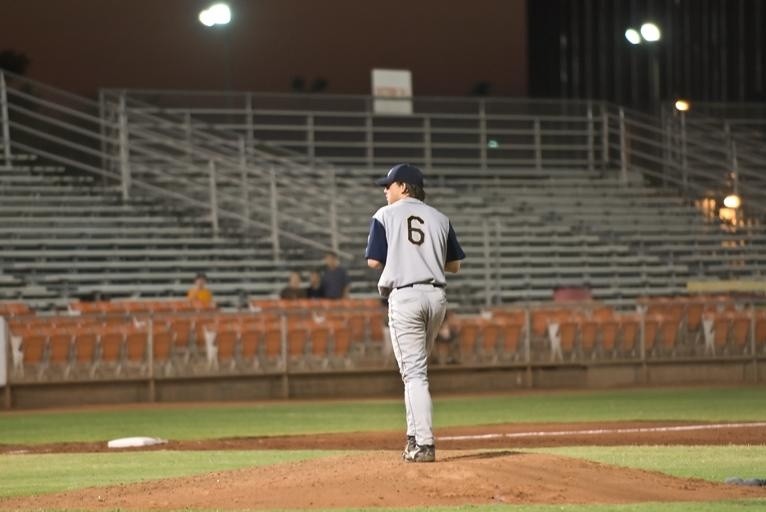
top-left (398, 282), bottom-right (443, 290)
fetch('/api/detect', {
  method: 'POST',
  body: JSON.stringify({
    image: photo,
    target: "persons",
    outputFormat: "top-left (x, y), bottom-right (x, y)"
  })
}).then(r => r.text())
top-left (188, 272), bottom-right (212, 301)
top-left (365, 163), bottom-right (465, 462)
top-left (279, 252), bottom-right (352, 299)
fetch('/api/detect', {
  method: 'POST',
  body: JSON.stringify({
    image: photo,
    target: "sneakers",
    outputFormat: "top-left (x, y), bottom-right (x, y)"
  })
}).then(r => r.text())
top-left (401, 434), bottom-right (436, 463)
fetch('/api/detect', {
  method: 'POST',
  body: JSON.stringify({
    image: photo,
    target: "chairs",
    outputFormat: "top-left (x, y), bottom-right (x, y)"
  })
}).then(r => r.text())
top-left (0, 292), bottom-right (766, 380)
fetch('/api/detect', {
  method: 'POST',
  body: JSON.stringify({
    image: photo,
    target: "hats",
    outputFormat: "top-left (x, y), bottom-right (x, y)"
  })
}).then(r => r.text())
top-left (374, 164), bottom-right (424, 188)
